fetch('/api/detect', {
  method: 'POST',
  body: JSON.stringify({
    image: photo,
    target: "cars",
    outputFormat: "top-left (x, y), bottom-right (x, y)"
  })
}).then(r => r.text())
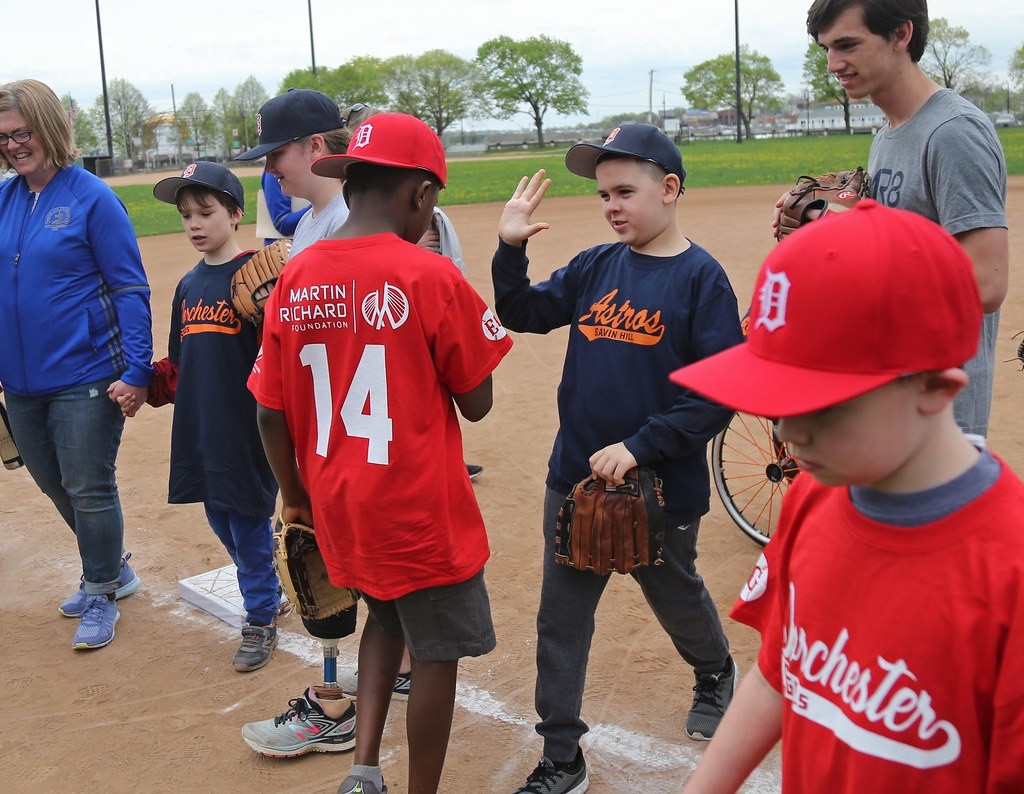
top-left (995, 113), bottom-right (1017, 127)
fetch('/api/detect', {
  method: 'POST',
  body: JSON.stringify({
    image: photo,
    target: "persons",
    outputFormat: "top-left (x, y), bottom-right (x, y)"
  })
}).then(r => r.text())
top-left (233, 89), bottom-right (514, 794)
top-left (663, 199), bottom-right (1024, 794)
top-left (108, 160), bottom-right (280, 673)
top-left (491, 124), bottom-right (745, 794)
top-left (0, 77), bottom-right (152, 648)
top-left (770, 0), bottom-right (1010, 443)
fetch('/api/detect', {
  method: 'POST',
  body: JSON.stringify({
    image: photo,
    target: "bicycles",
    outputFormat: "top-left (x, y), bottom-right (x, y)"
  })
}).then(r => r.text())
top-left (711, 412), bottom-right (800, 549)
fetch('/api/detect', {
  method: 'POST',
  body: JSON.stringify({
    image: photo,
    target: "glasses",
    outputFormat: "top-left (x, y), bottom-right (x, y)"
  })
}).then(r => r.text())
top-left (346, 102), bottom-right (370, 126)
top-left (0, 131), bottom-right (33, 143)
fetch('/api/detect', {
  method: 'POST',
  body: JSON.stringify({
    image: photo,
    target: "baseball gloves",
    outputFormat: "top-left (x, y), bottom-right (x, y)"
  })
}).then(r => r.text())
top-left (274, 518), bottom-right (364, 622)
top-left (230, 238), bottom-right (292, 318)
top-left (553, 464), bottom-right (668, 575)
top-left (778, 167), bottom-right (871, 245)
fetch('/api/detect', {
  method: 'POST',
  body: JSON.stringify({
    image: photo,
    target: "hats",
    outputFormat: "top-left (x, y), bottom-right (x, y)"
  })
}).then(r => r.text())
top-left (565, 124), bottom-right (688, 197)
top-left (311, 113), bottom-right (447, 189)
top-left (667, 198), bottom-right (985, 416)
top-left (234, 87), bottom-right (346, 161)
top-left (153, 160), bottom-right (244, 216)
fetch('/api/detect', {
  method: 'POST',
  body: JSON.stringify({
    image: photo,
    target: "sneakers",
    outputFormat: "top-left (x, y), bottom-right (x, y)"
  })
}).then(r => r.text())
top-left (336, 772), bottom-right (388, 794)
top-left (684, 655), bottom-right (740, 740)
top-left (513, 746), bottom-right (589, 794)
top-left (58, 552), bottom-right (140, 618)
top-left (241, 687), bottom-right (356, 758)
top-left (71, 595), bottom-right (120, 649)
top-left (233, 616), bottom-right (278, 671)
